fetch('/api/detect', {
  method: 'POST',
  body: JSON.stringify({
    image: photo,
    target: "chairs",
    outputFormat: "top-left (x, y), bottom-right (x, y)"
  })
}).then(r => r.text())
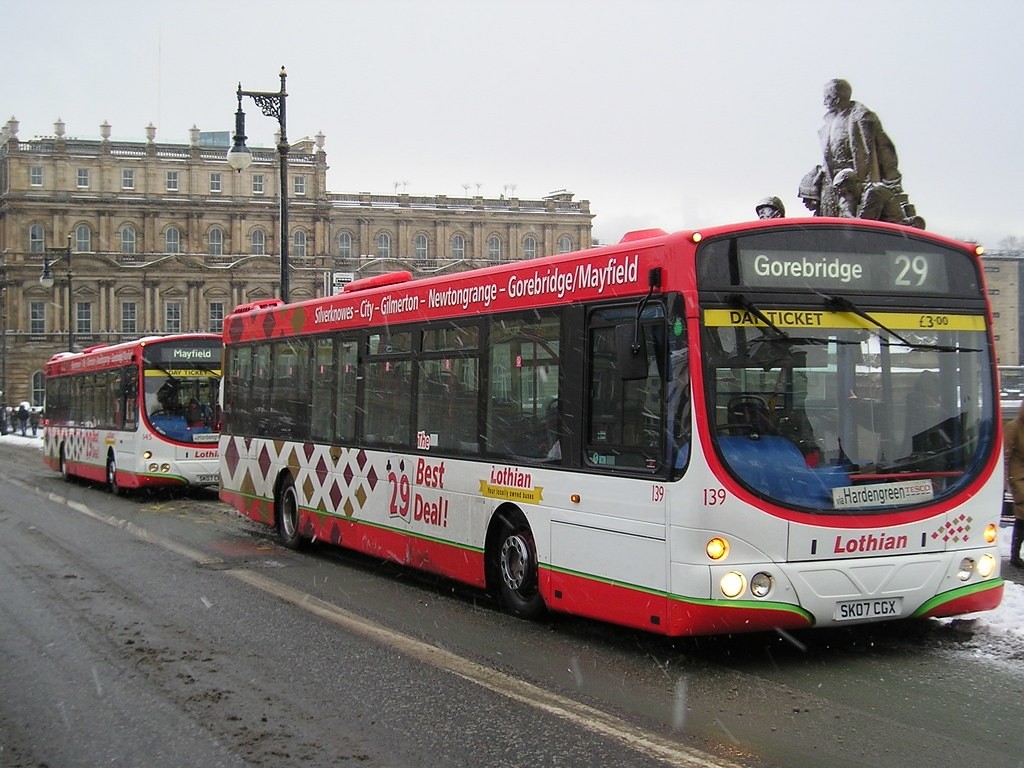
top-left (282, 363), bottom-right (559, 461)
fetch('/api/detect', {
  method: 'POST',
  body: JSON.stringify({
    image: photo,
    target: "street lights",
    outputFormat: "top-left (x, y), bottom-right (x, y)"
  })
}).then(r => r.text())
top-left (39, 236), bottom-right (75, 353)
top-left (225, 65), bottom-right (292, 306)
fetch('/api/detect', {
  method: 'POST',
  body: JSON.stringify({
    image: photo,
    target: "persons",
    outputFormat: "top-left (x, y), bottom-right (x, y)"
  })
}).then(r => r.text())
top-left (899, 370), bottom-right (939, 457)
top-left (1003, 398), bottom-right (1024, 569)
top-left (755, 78), bottom-right (906, 224)
top-left (10, 405), bottom-right (45, 438)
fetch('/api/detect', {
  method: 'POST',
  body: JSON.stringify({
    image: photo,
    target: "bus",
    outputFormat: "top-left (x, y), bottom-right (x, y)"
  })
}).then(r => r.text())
top-left (214, 216), bottom-right (1004, 638)
top-left (42, 331), bottom-right (224, 497)
